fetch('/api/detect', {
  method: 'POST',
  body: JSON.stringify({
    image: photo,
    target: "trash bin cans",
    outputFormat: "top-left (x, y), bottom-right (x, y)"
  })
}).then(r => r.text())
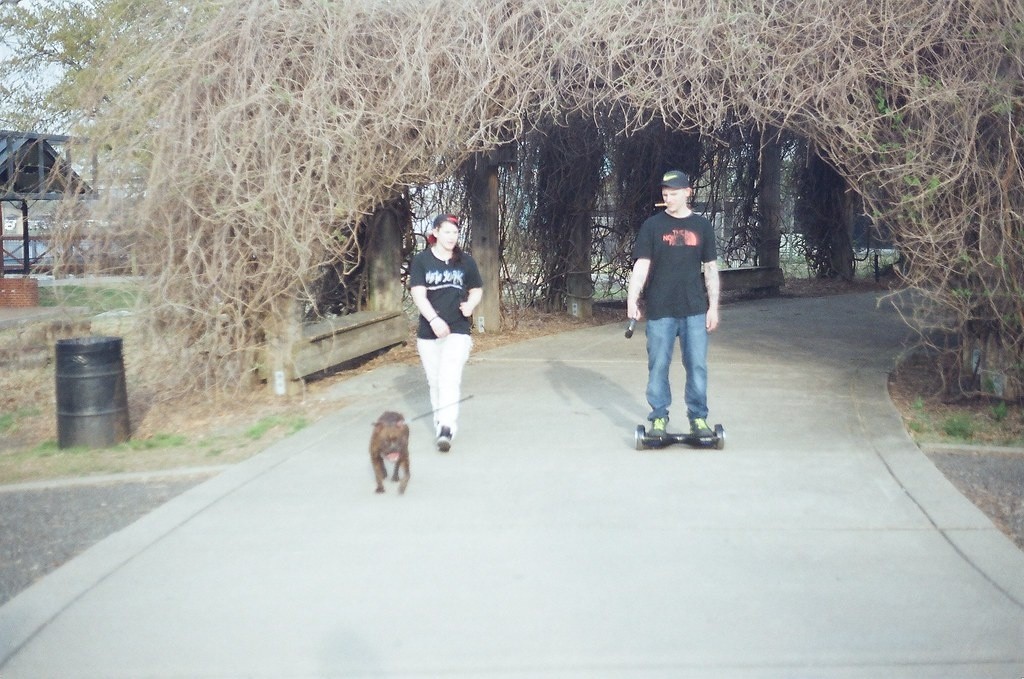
top-left (54, 334), bottom-right (132, 449)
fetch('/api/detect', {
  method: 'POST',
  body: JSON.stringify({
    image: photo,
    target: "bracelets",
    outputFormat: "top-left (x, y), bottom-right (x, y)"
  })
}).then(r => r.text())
top-left (429, 316), bottom-right (439, 323)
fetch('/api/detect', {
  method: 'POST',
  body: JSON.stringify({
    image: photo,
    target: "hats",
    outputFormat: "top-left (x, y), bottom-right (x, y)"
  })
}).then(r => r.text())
top-left (659, 171), bottom-right (690, 188)
top-left (428, 214), bottom-right (460, 244)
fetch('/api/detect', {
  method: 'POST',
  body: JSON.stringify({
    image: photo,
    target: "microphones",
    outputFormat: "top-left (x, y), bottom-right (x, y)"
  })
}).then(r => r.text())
top-left (625, 318), bottom-right (637, 339)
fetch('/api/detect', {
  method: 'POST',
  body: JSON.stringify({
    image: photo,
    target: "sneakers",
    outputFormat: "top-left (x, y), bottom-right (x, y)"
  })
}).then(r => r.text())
top-left (690, 417), bottom-right (713, 437)
top-left (649, 417), bottom-right (669, 437)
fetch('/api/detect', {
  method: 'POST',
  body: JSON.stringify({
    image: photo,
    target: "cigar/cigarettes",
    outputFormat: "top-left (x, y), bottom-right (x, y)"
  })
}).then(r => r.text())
top-left (655, 204), bottom-right (672, 206)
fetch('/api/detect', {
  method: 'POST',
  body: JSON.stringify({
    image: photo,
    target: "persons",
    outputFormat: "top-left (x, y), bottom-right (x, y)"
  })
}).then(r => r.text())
top-left (408, 214), bottom-right (483, 451)
top-left (628, 171), bottom-right (720, 436)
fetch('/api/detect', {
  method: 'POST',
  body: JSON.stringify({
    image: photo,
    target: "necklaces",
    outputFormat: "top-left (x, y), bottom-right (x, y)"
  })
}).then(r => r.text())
top-left (435, 246), bottom-right (450, 265)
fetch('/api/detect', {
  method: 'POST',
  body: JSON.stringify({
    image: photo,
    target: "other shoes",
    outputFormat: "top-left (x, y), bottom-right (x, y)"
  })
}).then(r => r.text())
top-left (437, 426), bottom-right (452, 452)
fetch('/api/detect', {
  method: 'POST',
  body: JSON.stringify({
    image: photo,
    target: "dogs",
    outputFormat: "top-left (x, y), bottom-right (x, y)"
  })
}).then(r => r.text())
top-left (369, 411), bottom-right (411, 495)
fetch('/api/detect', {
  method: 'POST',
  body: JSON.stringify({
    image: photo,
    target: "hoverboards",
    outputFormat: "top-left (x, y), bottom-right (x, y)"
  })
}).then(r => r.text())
top-left (636, 423), bottom-right (725, 450)
